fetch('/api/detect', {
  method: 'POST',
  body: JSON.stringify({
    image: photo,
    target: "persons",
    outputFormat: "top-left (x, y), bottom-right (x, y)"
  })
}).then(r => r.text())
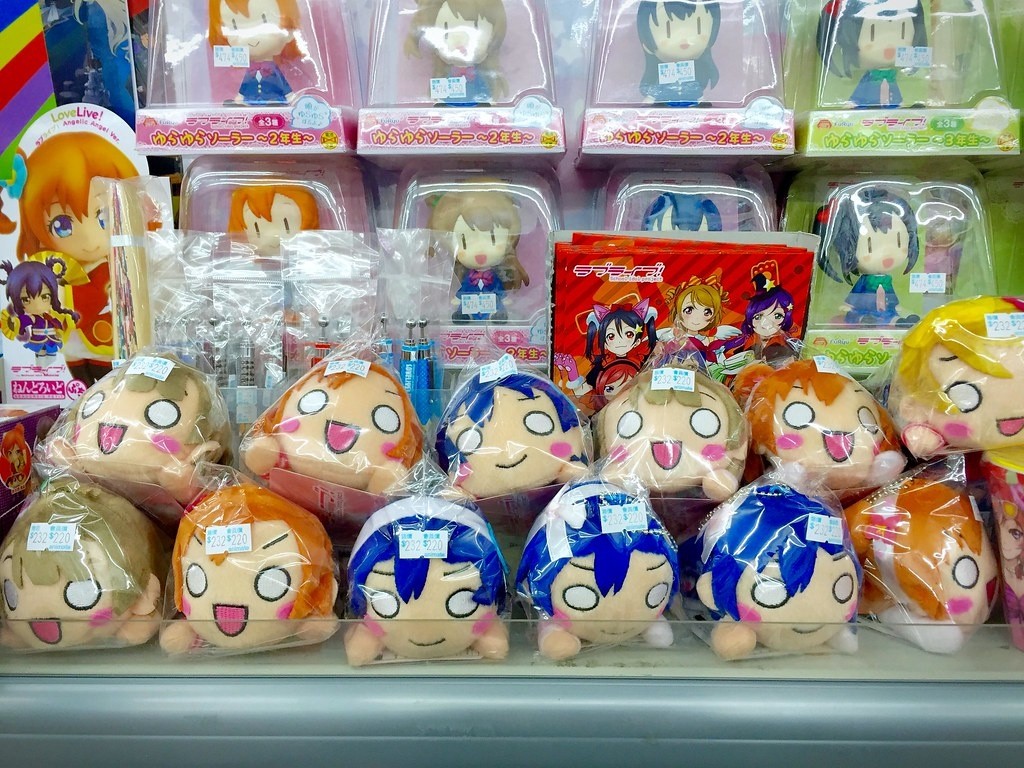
top-left (200, 0), bottom-right (966, 342)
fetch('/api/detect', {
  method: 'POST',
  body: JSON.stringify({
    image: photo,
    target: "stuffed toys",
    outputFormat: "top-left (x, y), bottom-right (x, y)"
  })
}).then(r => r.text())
top-left (835, 473), bottom-right (1000, 654)
top-left (158, 479), bottom-right (341, 660)
top-left (338, 494), bottom-right (511, 667)
top-left (1, 480), bottom-right (173, 655)
top-left (437, 359), bottom-right (590, 504)
top-left (242, 356), bottom-right (427, 521)
top-left (889, 298), bottom-right (1024, 507)
top-left (589, 364), bottom-right (752, 504)
top-left (725, 352), bottom-right (909, 499)
top-left (670, 477), bottom-right (863, 664)
top-left (511, 480), bottom-right (680, 662)
top-left (52, 354), bottom-right (235, 517)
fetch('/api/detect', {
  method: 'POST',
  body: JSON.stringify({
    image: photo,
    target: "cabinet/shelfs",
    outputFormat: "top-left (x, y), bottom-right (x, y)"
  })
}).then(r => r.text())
top-left (2, 621), bottom-right (1024, 768)
